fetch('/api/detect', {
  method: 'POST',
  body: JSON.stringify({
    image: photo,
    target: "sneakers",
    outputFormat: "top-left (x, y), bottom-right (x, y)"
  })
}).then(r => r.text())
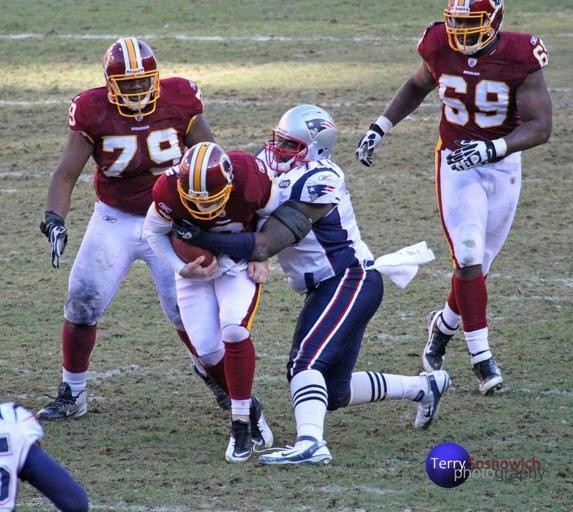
top-left (259, 438), bottom-right (332, 465)
top-left (225, 418), bottom-right (253, 464)
top-left (470, 350), bottom-right (502, 395)
top-left (421, 310), bottom-right (453, 371)
top-left (193, 364), bottom-right (230, 410)
top-left (423, 370), bottom-right (450, 429)
top-left (36, 383), bottom-right (89, 421)
top-left (249, 396), bottom-right (274, 453)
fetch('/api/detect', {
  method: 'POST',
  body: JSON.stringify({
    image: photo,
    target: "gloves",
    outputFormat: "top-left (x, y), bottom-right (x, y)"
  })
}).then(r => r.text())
top-left (172, 219), bottom-right (208, 244)
top-left (40, 210), bottom-right (69, 269)
top-left (446, 139), bottom-right (496, 172)
top-left (354, 123), bottom-right (384, 167)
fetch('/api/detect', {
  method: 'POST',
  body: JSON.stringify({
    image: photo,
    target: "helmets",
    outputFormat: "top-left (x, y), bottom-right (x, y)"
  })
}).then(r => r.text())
top-left (104, 37), bottom-right (161, 118)
top-left (177, 141), bottom-right (234, 220)
top-left (443, 1), bottom-right (504, 56)
top-left (264, 104), bottom-right (335, 174)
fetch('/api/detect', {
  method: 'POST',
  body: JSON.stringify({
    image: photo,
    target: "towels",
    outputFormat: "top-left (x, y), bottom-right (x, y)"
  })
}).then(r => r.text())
top-left (370, 240), bottom-right (435, 289)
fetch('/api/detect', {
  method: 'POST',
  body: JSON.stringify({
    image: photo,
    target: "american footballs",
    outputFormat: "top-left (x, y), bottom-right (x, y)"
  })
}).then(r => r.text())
top-left (170, 225), bottom-right (214, 270)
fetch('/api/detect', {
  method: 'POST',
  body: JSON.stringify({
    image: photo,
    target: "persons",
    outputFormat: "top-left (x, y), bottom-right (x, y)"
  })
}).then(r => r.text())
top-left (33, 37), bottom-right (257, 422)
top-left (0, 400), bottom-right (89, 512)
top-left (171, 101), bottom-right (451, 467)
top-left (144, 142), bottom-right (281, 464)
top-left (352, 0), bottom-right (554, 398)
